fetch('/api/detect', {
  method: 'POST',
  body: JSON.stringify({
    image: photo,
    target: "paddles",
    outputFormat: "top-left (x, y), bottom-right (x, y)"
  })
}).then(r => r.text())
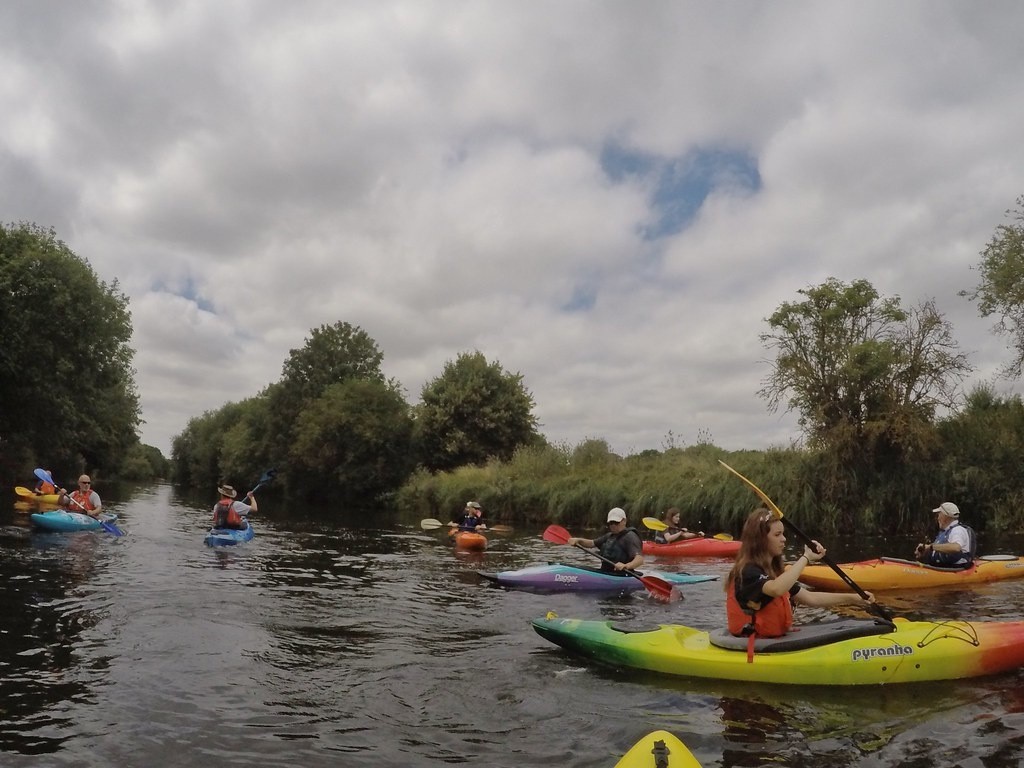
top-left (240, 469), bottom-right (276, 504)
top-left (543, 525), bottom-right (683, 599)
top-left (716, 456), bottom-right (913, 626)
top-left (421, 517), bottom-right (515, 538)
top-left (34, 469), bottom-right (125, 536)
top-left (642, 516), bottom-right (734, 542)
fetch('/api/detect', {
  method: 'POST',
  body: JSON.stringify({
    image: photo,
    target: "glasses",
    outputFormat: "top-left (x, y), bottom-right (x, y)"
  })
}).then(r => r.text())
top-left (80, 481), bottom-right (91, 484)
top-left (609, 518), bottom-right (624, 525)
top-left (473, 507), bottom-right (480, 510)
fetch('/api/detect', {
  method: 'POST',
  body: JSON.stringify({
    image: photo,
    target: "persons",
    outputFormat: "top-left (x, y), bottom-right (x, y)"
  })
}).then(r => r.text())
top-left (35, 471), bottom-right (60, 495)
top-left (915, 503), bottom-right (977, 565)
top-left (448, 501), bottom-right (486, 533)
top-left (724, 509), bottom-right (875, 638)
top-left (58, 475), bottom-right (102, 516)
top-left (569, 508), bottom-right (644, 572)
top-left (214, 485), bottom-right (257, 529)
top-left (655, 507), bottom-right (704, 544)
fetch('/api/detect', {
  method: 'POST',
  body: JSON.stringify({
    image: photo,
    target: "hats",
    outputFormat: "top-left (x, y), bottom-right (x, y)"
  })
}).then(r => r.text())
top-left (932, 502), bottom-right (960, 518)
top-left (465, 501), bottom-right (482, 510)
top-left (606, 507), bottom-right (626, 522)
top-left (217, 484), bottom-right (237, 498)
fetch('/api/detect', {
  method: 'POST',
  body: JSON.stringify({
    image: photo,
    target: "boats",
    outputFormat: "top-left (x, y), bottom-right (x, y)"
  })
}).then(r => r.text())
top-left (611, 730), bottom-right (703, 768)
top-left (640, 535), bottom-right (744, 556)
top-left (12, 486), bottom-right (117, 532)
top-left (526, 616), bottom-right (1024, 697)
top-left (448, 527), bottom-right (487, 551)
top-left (473, 562), bottom-right (720, 593)
top-left (203, 517), bottom-right (254, 547)
top-left (786, 555), bottom-right (1024, 592)
